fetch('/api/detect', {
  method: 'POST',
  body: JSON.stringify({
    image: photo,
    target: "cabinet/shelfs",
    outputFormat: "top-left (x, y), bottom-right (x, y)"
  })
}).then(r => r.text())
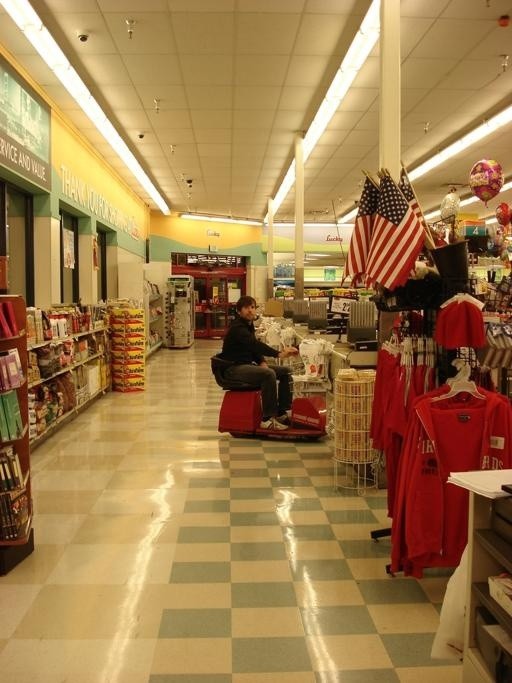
top-left (0, 293), bottom-right (35, 547)
top-left (461, 484), bottom-right (512, 682)
top-left (26, 325), bottom-right (112, 455)
top-left (148, 293), bottom-right (166, 355)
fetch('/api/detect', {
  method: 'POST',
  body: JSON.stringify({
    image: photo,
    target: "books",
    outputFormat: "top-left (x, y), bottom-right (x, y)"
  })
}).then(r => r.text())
top-left (0, 294), bottom-right (32, 540)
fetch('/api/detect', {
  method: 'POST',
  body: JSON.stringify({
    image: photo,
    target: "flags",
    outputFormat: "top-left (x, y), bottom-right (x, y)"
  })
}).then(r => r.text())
top-left (341, 161), bottom-right (437, 292)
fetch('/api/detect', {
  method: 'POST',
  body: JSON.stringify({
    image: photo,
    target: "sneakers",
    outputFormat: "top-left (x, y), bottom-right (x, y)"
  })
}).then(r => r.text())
top-left (260, 411), bottom-right (291, 431)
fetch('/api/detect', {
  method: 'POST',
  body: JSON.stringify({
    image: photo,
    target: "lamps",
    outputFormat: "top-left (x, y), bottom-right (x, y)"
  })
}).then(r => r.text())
top-left (179, 212), bottom-right (263, 226)
top-left (1, 0), bottom-right (173, 215)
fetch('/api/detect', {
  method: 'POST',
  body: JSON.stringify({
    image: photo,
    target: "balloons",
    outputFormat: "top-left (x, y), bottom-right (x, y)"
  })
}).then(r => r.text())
top-left (495, 202), bottom-right (511, 228)
top-left (468, 158), bottom-right (502, 204)
top-left (440, 192), bottom-right (462, 225)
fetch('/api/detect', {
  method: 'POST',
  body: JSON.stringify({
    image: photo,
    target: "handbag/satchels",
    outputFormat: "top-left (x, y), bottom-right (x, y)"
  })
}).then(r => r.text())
top-left (430, 544), bottom-right (467, 661)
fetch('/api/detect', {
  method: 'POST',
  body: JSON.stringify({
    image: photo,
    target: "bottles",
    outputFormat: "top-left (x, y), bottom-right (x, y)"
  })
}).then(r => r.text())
top-left (85, 305), bottom-right (91, 330)
top-left (42, 309), bottom-right (79, 338)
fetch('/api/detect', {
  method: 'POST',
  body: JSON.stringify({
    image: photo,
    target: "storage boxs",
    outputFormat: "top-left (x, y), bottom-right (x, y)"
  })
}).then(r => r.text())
top-left (111, 309), bottom-right (145, 391)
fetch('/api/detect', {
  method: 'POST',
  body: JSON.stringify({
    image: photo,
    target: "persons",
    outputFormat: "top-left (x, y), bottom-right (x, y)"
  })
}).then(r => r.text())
top-left (222, 295), bottom-right (299, 431)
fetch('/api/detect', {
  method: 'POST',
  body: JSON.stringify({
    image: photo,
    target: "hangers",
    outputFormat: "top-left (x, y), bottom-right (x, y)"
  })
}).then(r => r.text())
top-left (426, 360), bottom-right (487, 402)
top-left (379, 335), bottom-right (435, 404)
top-left (445, 356), bottom-right (465, 386)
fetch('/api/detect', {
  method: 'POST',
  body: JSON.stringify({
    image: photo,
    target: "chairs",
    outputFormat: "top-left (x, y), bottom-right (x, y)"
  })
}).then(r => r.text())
top-left (209, 349), bottom-right (265, 392)
